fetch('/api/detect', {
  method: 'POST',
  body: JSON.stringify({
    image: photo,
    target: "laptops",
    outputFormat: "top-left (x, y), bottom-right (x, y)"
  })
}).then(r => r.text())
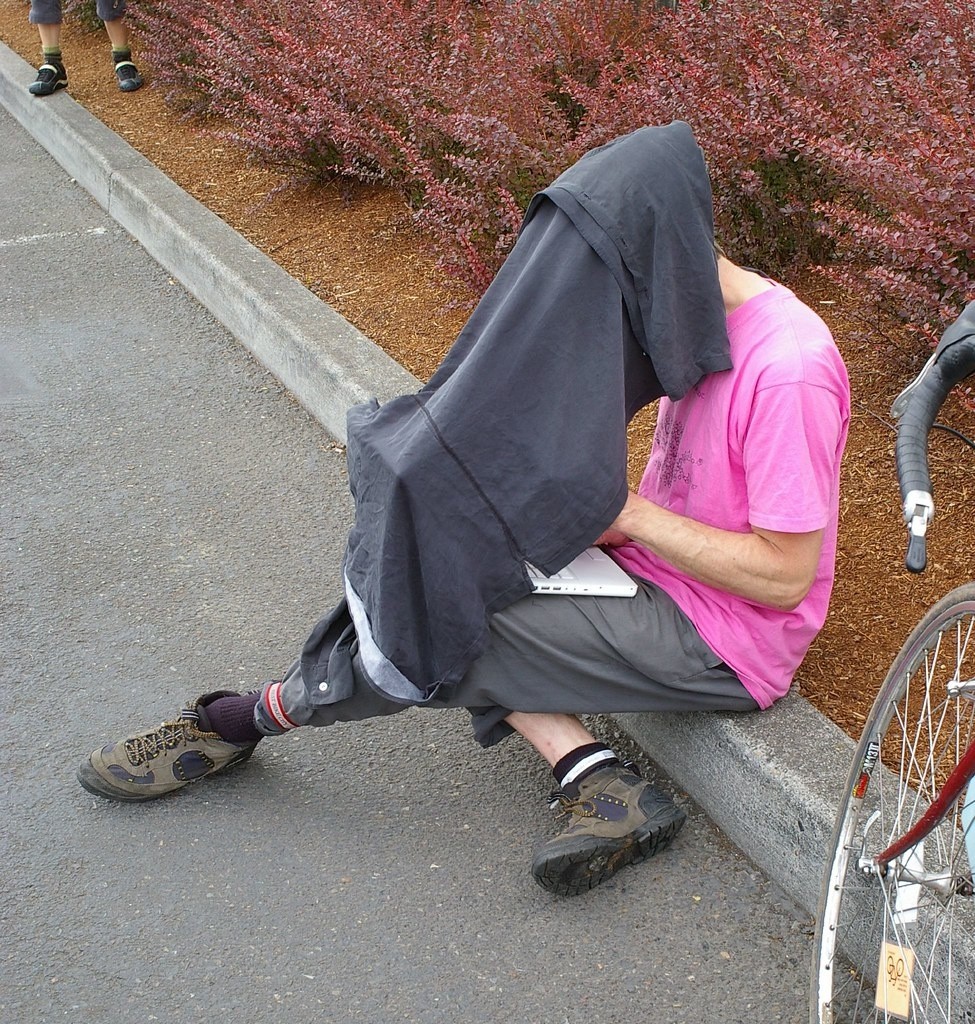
top-left (527, 546), bottom-right (638, 598)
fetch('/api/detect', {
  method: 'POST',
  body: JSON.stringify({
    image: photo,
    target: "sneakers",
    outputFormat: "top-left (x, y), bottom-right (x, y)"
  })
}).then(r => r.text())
top-left (77, 691), bottom-right (258, 802)
top-left (531, 761), bottom-right (685, 898)
top-left (114, 52), bottom-right (142, 92)
top-left (29, 61), bottom-right (68, 95)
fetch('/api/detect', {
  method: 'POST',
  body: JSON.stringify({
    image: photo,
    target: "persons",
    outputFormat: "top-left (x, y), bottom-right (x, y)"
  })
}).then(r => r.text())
top-left (29, 0), bottom-right (143, 96)
top-left (75, 121), bottom-right (851, 897)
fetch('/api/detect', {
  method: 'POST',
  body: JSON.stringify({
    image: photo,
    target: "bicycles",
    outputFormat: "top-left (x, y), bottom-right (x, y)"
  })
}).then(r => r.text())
top-left (810, 300), bottom-right (975, 1024)
